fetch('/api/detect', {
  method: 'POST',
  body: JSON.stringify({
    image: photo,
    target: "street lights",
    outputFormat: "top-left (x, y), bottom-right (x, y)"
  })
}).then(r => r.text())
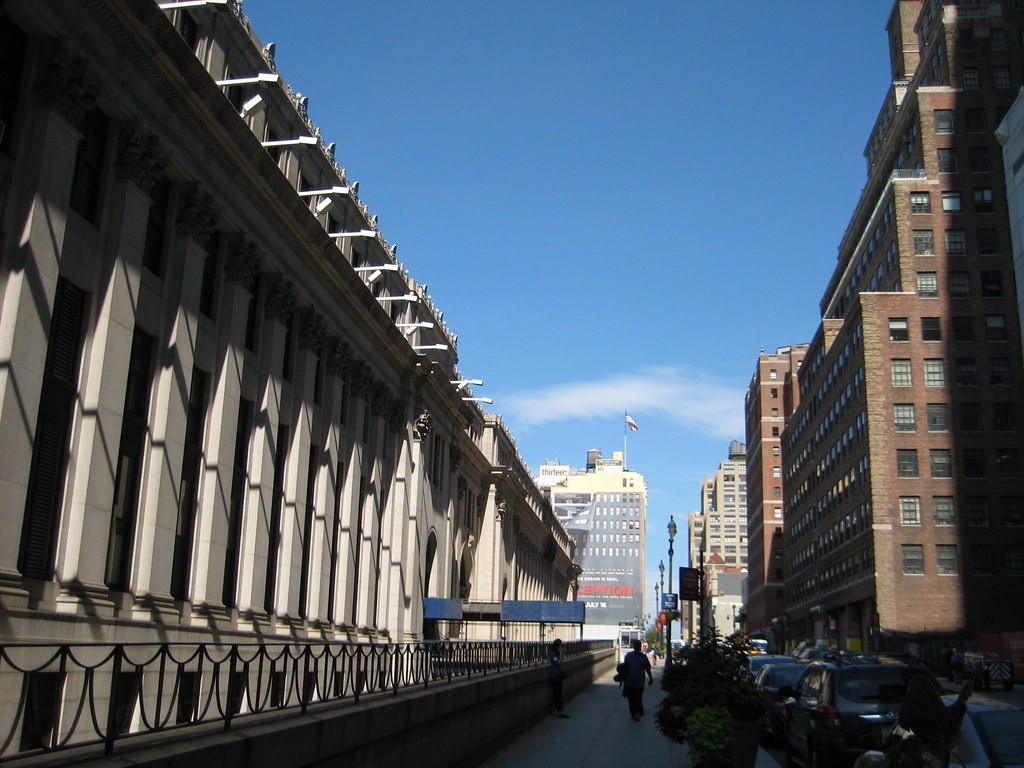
top-left (664, 515), bottom-right (677, 670)
top-left (654, 582), bottom-right (665, 659)
top-left (658, 560), bottom-right (667, 659)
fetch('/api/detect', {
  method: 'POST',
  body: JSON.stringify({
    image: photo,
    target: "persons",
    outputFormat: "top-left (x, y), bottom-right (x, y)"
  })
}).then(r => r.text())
top-left (623, 639), bottom-right (653, 721)
top-left (652, 640), bottom-right (660, 665)
top-left (549, 639), bottom-right (571, 719)
top-left (882, 641), bottom-right (977, 768)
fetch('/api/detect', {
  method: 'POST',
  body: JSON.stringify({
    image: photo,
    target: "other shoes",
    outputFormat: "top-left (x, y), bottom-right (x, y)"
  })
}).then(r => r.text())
top-left (630, 713), bottom-right (641, 721)
top-left (558, 712), bottom-right (569, 719)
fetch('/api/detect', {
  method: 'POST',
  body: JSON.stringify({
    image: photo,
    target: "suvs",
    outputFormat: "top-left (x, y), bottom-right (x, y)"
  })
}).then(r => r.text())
top-left (772, 652), bottom-right (952, 768)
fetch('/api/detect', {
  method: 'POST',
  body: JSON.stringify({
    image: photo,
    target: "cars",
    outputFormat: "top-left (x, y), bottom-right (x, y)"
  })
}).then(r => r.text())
top-left (746, 656), bottom-right (799, 685)
top-left (670, 637), bottom-right (769, 661)
top-left (753, 663), bottom-right (819, 748)
top-left (854, 693), bottom-right (1024, 768)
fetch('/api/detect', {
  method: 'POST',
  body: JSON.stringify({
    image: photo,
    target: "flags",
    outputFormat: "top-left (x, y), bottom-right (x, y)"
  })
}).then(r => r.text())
top-left (625, 410), bottom-right (639, 431)
